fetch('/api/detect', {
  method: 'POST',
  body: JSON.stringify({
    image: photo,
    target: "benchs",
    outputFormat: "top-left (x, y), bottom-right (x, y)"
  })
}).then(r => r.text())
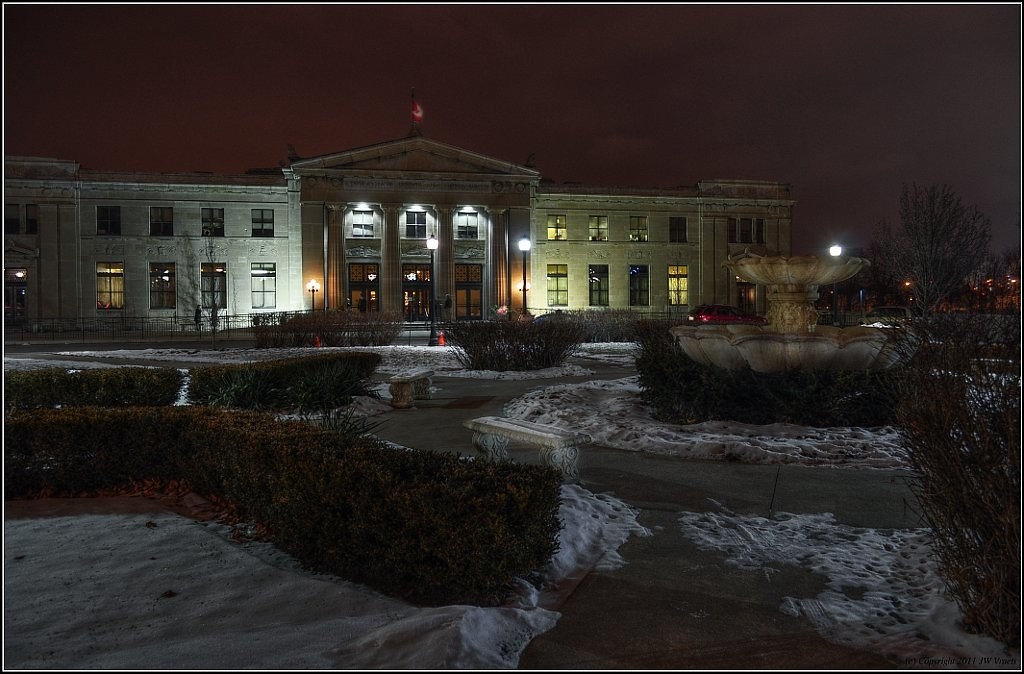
top-left (384, 368), bottom-right (435, 411)
top-left (463, 416), bottom-right (592, 484)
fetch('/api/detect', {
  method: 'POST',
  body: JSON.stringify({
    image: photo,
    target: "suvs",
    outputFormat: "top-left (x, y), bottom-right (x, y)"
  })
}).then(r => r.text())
top-left (685, 303), bottom-right (770, 328)
top-left (855, 304), bottom-right (915, 330)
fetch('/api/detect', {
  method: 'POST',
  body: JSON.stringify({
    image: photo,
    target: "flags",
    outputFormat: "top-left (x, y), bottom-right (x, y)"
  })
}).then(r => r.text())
top-left (411, 100), bottom-right (423, 121)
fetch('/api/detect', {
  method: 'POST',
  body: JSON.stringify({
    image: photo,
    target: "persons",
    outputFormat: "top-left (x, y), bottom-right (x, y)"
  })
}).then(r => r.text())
top-left (211, 304), bottom-right (217, 329)
top-left (358, 293), bottom-right (366, 313)
top-left (194, 305), bottom-right (201, 331)
top-left (444, 294), bottom-right (452, 323)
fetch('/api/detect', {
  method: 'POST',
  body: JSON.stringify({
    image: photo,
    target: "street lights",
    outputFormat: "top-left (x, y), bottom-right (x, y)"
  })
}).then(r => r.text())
top-left (425, 232), bottom-right (439, 346)
top-left (518, 235), bottom-right (532, 315)
top-left (307, 279), bottom-right (321, 312)
top-left (829, 245), bottom-right (842, 327)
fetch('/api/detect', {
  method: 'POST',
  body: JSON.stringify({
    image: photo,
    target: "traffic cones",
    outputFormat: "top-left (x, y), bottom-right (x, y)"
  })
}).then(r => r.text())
top-left (438, 328), bottom-right (446, 346)
